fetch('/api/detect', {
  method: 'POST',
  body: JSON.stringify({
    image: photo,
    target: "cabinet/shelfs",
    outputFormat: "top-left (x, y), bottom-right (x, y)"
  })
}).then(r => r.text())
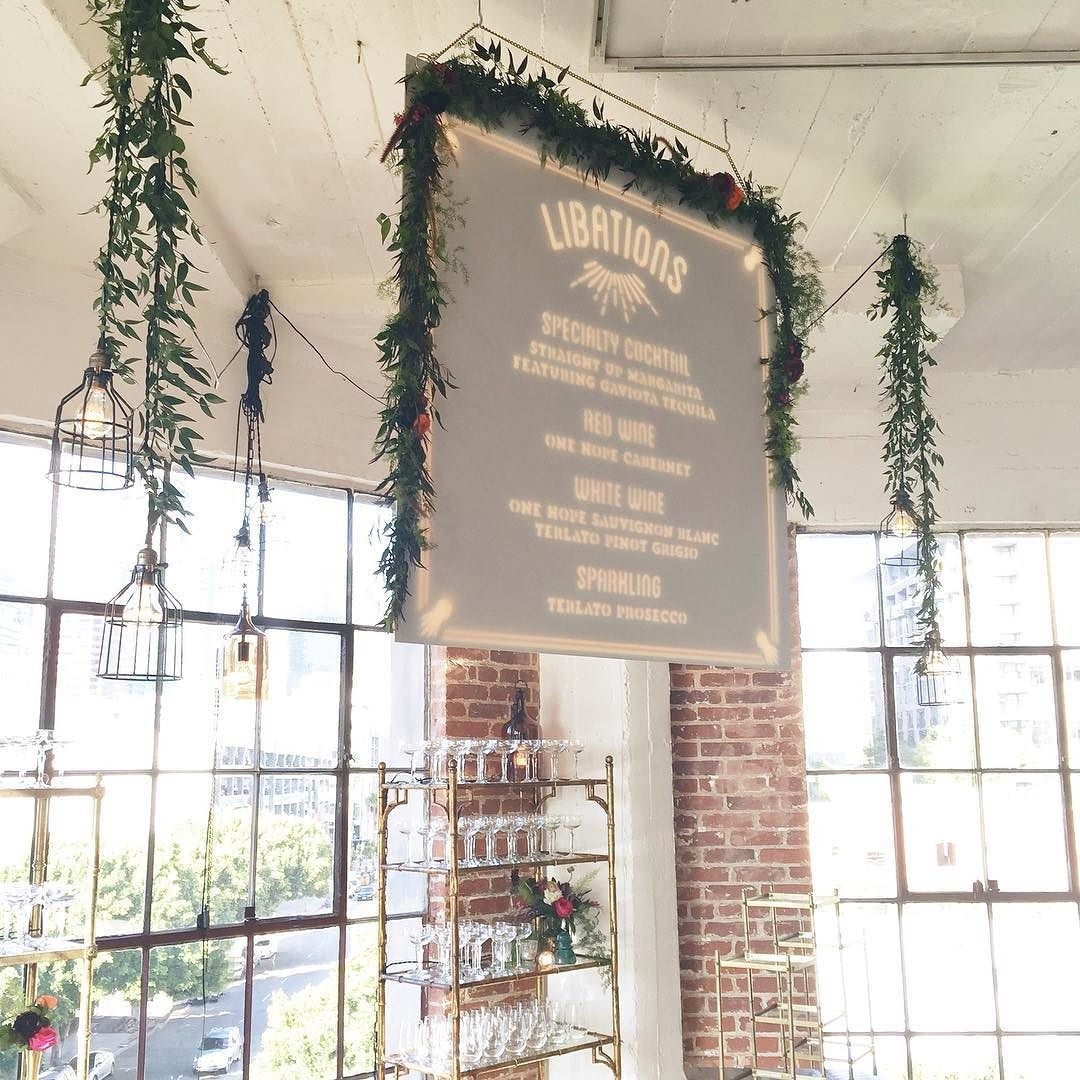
top-left (0, 773), bottom-right (107, 1080)
top-left (710, 948), bottom-right (826, 1080)
top-left (379, 757), bottom-right (623, 1078)
top-left (741, 881), bottom-right (878, 1080)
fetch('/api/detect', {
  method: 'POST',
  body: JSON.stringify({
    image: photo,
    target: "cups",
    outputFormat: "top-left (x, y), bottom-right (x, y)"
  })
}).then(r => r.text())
top-left (398, 999), bottom-right (586, 1064)
top-left (519, 941), bottom-right (537, 960)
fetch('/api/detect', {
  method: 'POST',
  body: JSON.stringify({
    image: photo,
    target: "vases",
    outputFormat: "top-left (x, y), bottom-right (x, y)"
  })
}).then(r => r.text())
top-left (551, 925), bottom-right (578, 966)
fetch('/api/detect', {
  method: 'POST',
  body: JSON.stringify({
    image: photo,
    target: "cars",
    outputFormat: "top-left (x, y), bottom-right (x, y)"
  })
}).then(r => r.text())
top-left (226, 937), bottom-right (279, 980)
top-left (356, 886), bottom-right (375, 902)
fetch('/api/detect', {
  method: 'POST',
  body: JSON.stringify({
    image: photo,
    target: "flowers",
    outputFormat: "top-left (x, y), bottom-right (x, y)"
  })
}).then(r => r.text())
top-left (516, 872), bottom-right (616, 992)
top-left (0, 995), bottom-right (58, 1055)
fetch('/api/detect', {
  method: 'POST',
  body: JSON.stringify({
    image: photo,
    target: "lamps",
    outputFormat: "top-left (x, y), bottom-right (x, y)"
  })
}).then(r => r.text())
top-left (912, 351), bottom-right (961, 708)
top-left (95, 0), bottom-right (232, 685)
top-left (45, 0), bottom-right (136, 494)
top-left (880, 233), bottom-right (927, 568)
top-left (216, 294), bottom-right (282, 695)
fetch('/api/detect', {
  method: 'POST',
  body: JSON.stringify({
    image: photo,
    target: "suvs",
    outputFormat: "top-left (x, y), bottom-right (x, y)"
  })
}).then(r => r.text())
top-left (192, 1026), bottom-right (243, 1073)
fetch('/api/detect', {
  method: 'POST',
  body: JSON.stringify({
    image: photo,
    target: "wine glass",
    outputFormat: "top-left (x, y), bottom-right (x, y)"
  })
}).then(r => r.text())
top-left (0, 882), bottom-right (77, 953)
top-left (404, 920), bottom-right (531, 986)
top-left (397, 813), bottom-right (581, 868)
top-left (400, 738), bottom-right (584, 781)
top-left (0, 728), bottom-right (55, 788)
